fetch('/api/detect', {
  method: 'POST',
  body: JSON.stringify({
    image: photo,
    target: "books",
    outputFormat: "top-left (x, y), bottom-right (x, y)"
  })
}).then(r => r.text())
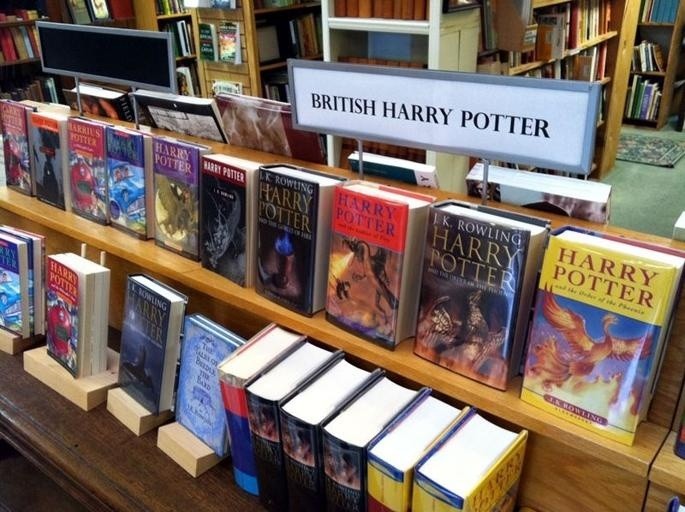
top-left (0, 0), bottom-right (685, 510)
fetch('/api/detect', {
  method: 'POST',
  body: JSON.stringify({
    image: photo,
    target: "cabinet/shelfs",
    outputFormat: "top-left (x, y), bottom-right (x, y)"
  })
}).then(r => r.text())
top-left (0, 4), bottom-right (66, 118)
top-left (0, 108), bottom-right (684, 512)
top-left (474, 2), bottom-right (625, 188)
top-left (630, 5), bottom-right (685, 126)
top-left (155, 4), bottom-right (199, 96)
top-left (246, 4), bottom-right (324, 101)
top-left (320, 4), bottom-right (471, 197)
